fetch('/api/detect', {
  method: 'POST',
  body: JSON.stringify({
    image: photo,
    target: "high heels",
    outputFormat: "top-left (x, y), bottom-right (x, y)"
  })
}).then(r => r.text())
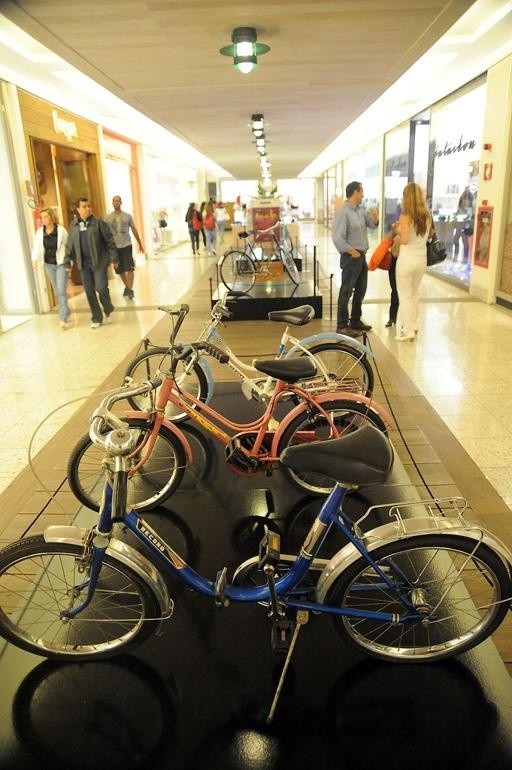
top-left (386, 319), bottom-right (396, 326)
top-left (414, 326), bottom-right (419, 336)
top-left (395, 332), bottom-right (415, 342)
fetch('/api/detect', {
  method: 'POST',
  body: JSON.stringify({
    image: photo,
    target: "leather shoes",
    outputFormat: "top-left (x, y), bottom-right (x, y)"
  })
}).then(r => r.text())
top-left (336, 327), bottom-right (362, 337)
top-left (349, 322), bottom-right (372, 331)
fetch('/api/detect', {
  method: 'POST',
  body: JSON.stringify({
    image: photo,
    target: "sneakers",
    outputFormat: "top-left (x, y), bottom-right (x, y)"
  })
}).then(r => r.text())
top-left (128, 290), bottom-right (133, 299)
top-left (92, 323), bottom-right (101, 328)
top-left (60, 321), bottom-right (73, 330)
top-left (123, 288), bottom-right (128, 296)
top-left (106, 311), bottom-right (114, 322)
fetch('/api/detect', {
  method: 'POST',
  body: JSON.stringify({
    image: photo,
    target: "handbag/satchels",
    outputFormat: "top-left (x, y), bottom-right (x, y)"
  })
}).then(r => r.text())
top-left (378, 251), bottom-right (392, 270)
top-left (367, 234), bottom-right (392, 271)
top-left (426, 238), bottom-right (446, 266)
top-left (193, 211), bottom-right (203, 230)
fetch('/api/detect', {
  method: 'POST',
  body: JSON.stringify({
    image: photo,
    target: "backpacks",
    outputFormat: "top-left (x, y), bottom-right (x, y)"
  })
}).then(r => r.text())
top-left (205, 213), bottom-right (215, 231)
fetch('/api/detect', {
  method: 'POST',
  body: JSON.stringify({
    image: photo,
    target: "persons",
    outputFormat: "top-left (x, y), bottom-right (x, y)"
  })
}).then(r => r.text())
top-left (233, 195), bottom-right (244, 223)
top-left (64, 198), bottom-right (119, 327)
top-left (205, 200), bottom-right (217, 256)
top-left (394, 183), bottom-right (434, 341)
top-left (213, 201), bottom-right (226, 242)
top-left (103, 194), bottom-right (145, 299)
top-left (185, 202), bottom-right (200, 254)
top-left (452, 184), bottom-right (476, 265)
top-left (198, 202), bottom-right (207, 246)
top-left (331, 181), bottom-right (379, 337)
top-left (32, 207), bottom-right (74, 329)
top-left (384, 214), bottom-right (400, 328)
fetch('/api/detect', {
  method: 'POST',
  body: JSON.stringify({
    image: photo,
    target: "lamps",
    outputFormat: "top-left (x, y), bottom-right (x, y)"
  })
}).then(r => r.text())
top-left (250, 115), bottom-right (264, 137)
top-left (252, 134), bottom-right (269, 152)
top-left (219, 27), bottom-right (270, 74)
